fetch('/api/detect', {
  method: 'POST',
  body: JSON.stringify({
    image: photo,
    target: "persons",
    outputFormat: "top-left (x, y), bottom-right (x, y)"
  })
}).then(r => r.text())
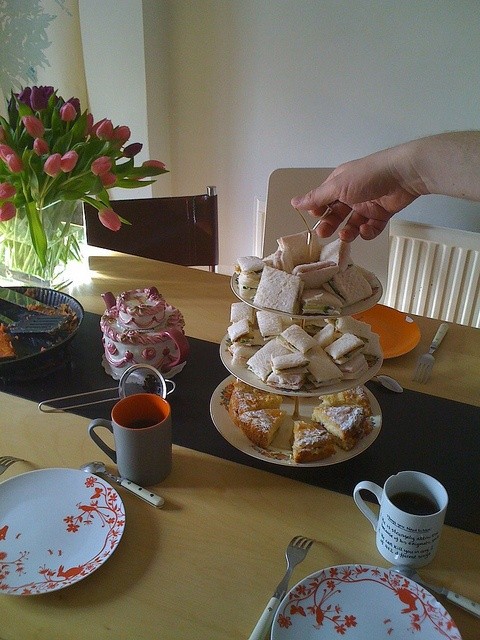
top-left (289, 131), bottom-right (480, 242)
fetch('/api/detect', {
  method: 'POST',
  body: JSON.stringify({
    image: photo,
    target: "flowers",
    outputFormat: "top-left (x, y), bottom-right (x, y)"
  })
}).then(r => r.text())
top-left (0, 87), bottom-right (167, 277)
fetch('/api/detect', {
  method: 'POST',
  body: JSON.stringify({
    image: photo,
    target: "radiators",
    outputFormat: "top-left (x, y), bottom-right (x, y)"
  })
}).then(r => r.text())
top-left (253, 199), bottom-right (479, 328)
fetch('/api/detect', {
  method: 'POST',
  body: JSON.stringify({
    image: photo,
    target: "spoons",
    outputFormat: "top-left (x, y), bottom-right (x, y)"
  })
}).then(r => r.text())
top-left (387, 566), bottom-right (480, 618)
top-left (370, 375), bottom-right (404, 393)
top-left (79, 462), bottom-right (166, 509)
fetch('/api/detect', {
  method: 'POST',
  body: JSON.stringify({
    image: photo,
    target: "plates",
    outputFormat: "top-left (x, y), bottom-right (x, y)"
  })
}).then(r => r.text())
top-left (219, 318), bottom-right (384, 398)
top-left (1, 286), bottom-right (85, 379)
top-left (231, 266), bottom-right (385, 321)
top-left (209, 376), bottom-right (384, 468)
top-left (270, 563), bottom-right (462, 640)
top-left (346, 304), bottom-right (421, 360)
top-left (0, 468), bottom-right (126, 597)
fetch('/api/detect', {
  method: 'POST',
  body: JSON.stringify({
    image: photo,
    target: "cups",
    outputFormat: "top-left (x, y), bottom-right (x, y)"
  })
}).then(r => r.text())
top-left (353, 470), bottom-right (450, 569)
top-left (88, 393), bottom-right (173, 486)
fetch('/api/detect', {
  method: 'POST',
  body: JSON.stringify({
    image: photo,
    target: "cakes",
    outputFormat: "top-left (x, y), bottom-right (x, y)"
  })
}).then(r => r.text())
top-left (224, 380), bottom-right (284, 428)
top-left (290, 416), bottom-right (335, 468)
top-left (319, 387), bottom-right (374, 417)
top-left (313, 403), bottom-right (366, 451)
top-left (234, 407), bottom-right (288, 450)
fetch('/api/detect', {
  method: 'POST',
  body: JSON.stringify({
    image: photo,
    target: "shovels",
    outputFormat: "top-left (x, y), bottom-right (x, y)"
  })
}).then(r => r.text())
top-left (0, 301), bottom-right (71, 335)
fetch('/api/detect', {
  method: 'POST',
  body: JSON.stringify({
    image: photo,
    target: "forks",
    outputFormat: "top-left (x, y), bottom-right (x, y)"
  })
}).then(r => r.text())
top-left (412, 323), bottom-right (450, 385)
top-left (0, 457), bottom-right (24, 476)
top-left (249, 536), bottom-right (316, 640)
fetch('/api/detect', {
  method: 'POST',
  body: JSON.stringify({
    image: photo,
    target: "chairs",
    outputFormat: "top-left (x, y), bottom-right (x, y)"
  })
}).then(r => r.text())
top-left (262, 166), bottom-right (390, 304)
top-left (79, 187), bottom-right (219, 274)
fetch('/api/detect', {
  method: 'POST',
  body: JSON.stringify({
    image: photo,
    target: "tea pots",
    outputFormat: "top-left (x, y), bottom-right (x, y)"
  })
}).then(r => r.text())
top-left (99, 286), bottom-right (190, 384)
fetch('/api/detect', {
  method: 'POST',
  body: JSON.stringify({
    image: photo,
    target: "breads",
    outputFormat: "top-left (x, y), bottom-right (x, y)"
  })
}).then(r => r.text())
top-left (225, 297), bottom-right (382, 393)
top-left (232, 224), bottom-right (379, 317)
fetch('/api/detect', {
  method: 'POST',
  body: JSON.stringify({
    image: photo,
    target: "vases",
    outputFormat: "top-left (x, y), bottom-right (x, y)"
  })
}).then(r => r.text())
top-left (5, 199), bottom-right (89, 291)
